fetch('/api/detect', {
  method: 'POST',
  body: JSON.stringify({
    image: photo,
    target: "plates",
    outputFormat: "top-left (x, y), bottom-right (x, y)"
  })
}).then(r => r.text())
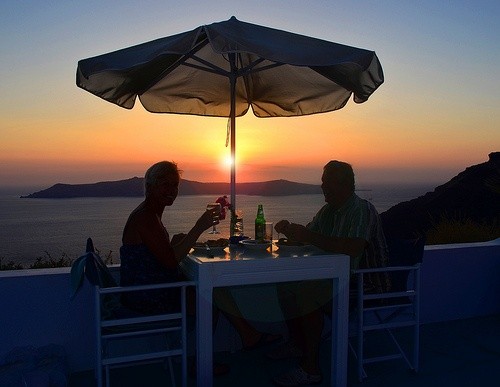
top-left (276, 243), bottom-right (311, 249)
top-left (193, 245), bottom-right (227, 250)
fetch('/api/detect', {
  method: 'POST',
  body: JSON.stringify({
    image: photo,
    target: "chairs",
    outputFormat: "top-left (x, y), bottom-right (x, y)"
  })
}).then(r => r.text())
top-left (351, 227), bottom-right (424, 383)
top-left (83, 239), bottom-right (196, 387)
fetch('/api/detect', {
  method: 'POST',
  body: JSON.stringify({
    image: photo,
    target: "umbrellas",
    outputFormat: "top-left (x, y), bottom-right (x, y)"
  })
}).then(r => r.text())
top-left (76, 16), bottom-right (385, 238)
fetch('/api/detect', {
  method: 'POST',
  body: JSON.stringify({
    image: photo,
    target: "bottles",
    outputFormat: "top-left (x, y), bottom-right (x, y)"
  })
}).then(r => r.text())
top-left (255, 204), bottom-right (266, 240)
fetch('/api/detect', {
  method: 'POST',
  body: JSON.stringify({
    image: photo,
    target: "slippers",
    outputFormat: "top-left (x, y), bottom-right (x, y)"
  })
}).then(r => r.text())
top-left (271, 367), bottom-right (322, 387)
top-left (190, 360), bottom-right (231, 376)
top-left (242, 332), bottom-right (284, 352)
top-left (264, 345), bottom-right (305, 360)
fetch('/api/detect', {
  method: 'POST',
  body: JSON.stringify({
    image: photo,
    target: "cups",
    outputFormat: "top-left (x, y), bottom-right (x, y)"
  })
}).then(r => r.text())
top-left (280, 220), bottom-right (291, 242)
top-left (264, 223), bottom-right (273, 244)
top-left (232, 209), bottom-right (243, 237)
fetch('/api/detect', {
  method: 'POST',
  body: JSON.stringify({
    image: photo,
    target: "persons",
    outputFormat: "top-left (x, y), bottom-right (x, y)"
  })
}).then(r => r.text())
top-left (267, 161), bottom-right (390, 387)
top-left (120, 161), bottom-right (283, 376)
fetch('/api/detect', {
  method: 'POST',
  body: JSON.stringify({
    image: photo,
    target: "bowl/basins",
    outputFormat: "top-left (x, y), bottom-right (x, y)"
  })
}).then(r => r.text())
top-left (239, 240), bottom-right (272, 251)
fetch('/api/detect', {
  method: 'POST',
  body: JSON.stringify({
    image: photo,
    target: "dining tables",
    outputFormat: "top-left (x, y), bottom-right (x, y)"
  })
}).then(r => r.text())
top-left (187, 240), bottom-right (349, 387)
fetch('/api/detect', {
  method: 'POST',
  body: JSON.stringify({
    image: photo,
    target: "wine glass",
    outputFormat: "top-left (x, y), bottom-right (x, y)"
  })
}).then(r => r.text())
top-left (206, 203), bottom-right (221, 234)
